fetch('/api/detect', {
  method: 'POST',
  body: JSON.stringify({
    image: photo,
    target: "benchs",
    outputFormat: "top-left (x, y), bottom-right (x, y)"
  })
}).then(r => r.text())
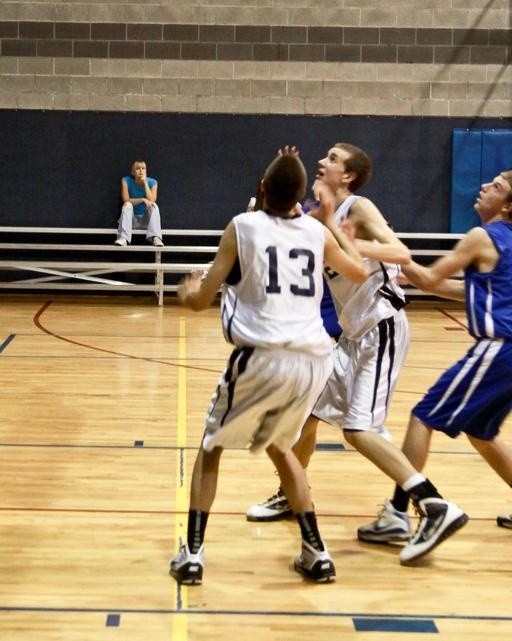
top-left (0, 225), bottom-right (469, 307)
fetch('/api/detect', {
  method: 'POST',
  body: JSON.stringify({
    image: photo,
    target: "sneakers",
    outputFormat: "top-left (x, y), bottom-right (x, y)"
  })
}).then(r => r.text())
top-left (153, 237), bottom-right (164, 247)
top-left (171, 543), bottom-right (204, 585)
top-left (115, 239), bottom-right (127, 246)
top-left (294, 542), bottom-right (337, 582)
top-left (399, 497), bottom-right (469, 563)
top-left (357, 500), bottom-right (413, 542)
top-left (246, 489), bottom-right (293, 522)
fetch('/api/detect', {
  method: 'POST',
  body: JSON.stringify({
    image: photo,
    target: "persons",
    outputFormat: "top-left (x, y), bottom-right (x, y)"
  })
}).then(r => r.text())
top-left (244, 143), bottom-right (470, 566)
top-left (357, 171), bottom-right (512, 543)
top-left (114, 159), bottom-right (164, 247)
top-left (168, 154), bottom-right (368, 587)
top-left (246, 182), bottom-right (343, 346)
